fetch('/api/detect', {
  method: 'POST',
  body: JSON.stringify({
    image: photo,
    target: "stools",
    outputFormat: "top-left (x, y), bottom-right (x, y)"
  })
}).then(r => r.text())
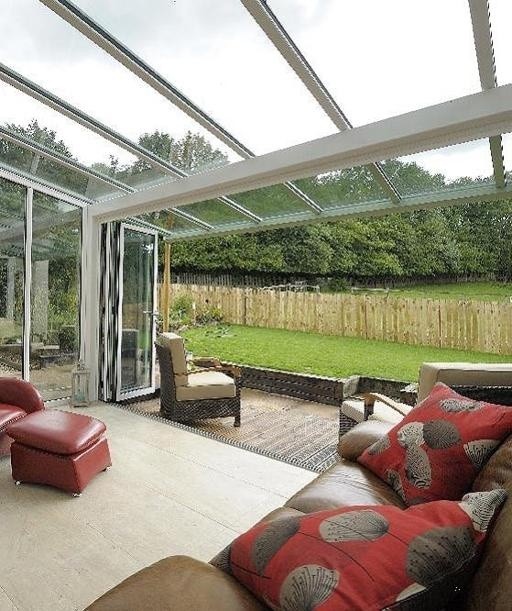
top-left (5, 410), bottom-right (112, 498)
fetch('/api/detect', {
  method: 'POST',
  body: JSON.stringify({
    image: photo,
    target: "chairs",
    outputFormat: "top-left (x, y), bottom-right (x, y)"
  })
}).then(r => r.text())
top-left (154, 332), bottom-right (243, 427)
top-left (0, 376), bottom-right (44, 457)
top-left (340, 362), bottom-right (512, 437)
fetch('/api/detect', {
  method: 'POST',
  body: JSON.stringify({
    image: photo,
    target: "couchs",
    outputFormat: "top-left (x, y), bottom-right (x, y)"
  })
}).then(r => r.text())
top-left (82, 419), bottom-right (512, 611)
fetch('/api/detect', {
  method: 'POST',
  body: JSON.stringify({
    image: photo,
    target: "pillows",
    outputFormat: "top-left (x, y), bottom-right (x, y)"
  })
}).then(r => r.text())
top-left (209, 487), bottom-right (508, 610)
top-left (358, 382), bottom-right (512, 508)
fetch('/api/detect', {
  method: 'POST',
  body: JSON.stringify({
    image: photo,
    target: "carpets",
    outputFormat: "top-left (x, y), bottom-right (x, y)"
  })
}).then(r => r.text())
top-left (0, 401), bottom-right (325, 611)
